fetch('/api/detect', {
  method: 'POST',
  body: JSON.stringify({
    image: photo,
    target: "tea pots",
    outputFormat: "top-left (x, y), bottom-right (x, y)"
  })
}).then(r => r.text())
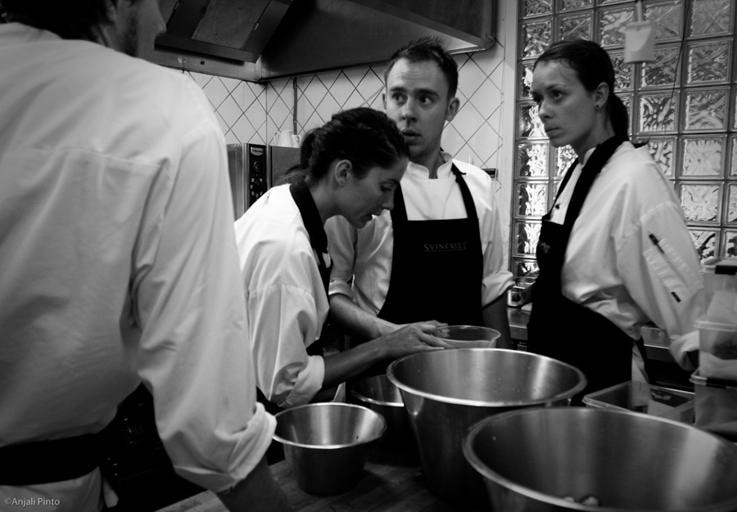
top-left (274, 128), bottom-right (302, 148)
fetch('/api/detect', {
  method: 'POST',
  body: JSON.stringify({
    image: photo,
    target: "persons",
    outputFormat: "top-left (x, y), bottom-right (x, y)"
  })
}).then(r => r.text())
top-left (0, 0), bottom-right (289, 512)
top-left (528, 37), bottom-right (703, 406)
top-left (321, 37), bottom-right (512, 377)
top-left (234, 106), bottom-right (447, 410)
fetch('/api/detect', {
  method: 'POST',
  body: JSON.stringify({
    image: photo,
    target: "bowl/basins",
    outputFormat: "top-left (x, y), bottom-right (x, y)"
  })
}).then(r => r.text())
top-left (387, 346), bottom-right (588, 486)
top-left (459, 408), bottom-right (737, 511)
top-left (355, 375), bottom-right (415, 463)
top-left (271, 402), bottom-right (384, 495)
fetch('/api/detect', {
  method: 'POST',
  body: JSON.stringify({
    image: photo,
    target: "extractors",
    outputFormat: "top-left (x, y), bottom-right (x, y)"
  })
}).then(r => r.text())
top-left (95, 1), bottom-right (500, 87)
